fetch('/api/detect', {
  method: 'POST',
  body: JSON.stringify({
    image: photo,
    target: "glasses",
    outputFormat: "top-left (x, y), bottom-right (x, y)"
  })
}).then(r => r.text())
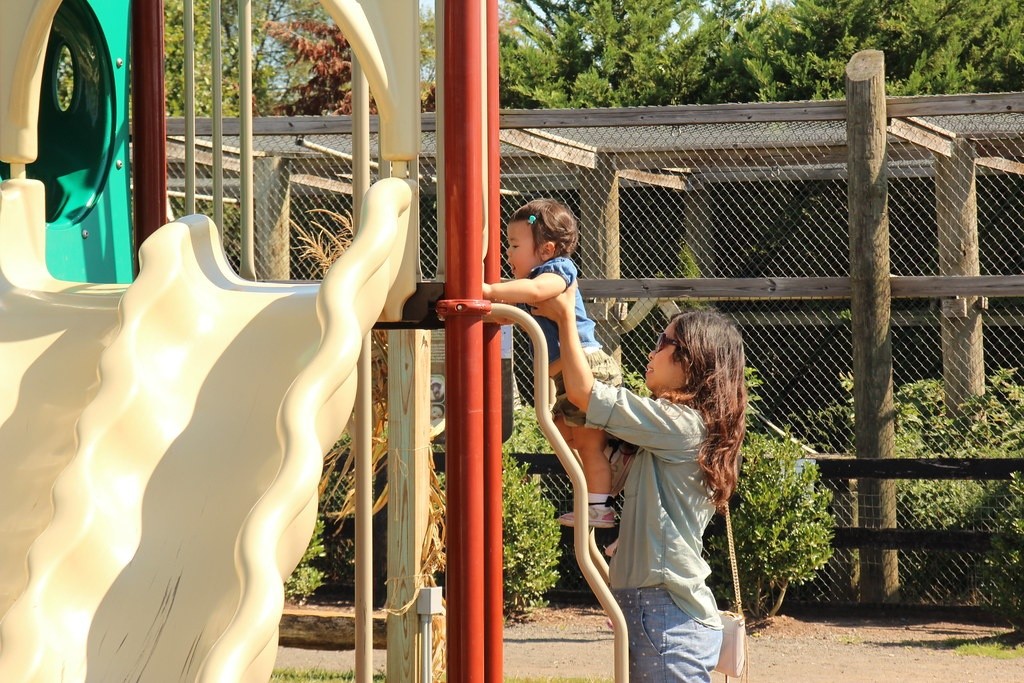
top-left (656, 333), bottom-right (688, 352)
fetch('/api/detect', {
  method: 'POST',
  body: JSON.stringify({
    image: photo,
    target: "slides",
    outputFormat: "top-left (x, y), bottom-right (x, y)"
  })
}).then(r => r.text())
top-left (0, 173), bottom-right (416, 682)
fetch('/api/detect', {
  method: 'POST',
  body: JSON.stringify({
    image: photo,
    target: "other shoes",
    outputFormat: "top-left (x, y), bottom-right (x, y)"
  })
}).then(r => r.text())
top-left (557, 505), bottom-right (616, 528)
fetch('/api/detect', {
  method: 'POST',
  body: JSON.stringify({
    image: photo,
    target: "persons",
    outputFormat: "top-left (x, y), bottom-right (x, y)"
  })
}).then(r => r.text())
top-left (525, 278), bottom-right (746, 683)
top-left (483, 199), bottom-right (622, 528)
top-left (429, 375), bottom-right (445, 429)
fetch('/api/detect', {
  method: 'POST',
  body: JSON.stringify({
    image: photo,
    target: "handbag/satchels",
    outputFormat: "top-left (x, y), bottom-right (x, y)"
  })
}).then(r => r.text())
top-left (712, 610), bottom-right (746, 678)
